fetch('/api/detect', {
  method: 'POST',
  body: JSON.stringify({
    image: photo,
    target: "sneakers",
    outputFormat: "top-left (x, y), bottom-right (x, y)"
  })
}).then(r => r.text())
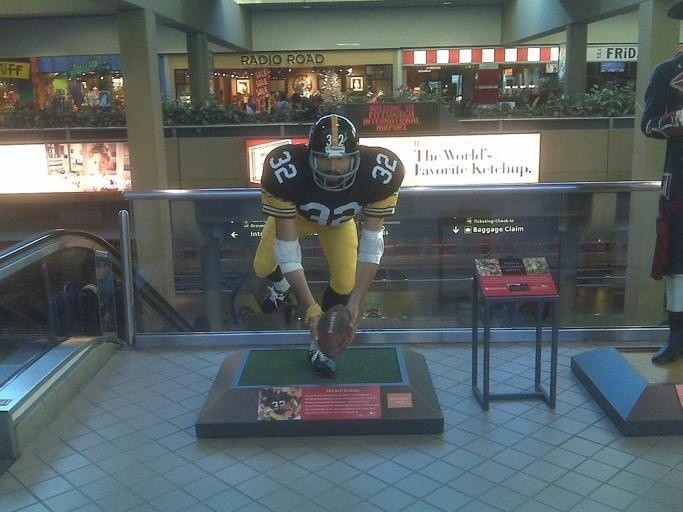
top-left (307, 350), bottom-right (335, 376)
top-left (260, 284), bottom-right (291, 313)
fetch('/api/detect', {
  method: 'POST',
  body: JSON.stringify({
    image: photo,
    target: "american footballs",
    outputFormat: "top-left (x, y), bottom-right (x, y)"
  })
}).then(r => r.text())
top-left (316, 303), bottom-right (351, 357)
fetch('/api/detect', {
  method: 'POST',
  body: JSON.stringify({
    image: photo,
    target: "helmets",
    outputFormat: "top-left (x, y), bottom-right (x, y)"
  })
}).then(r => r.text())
top-left (307, 114), bottom-right (359, 192)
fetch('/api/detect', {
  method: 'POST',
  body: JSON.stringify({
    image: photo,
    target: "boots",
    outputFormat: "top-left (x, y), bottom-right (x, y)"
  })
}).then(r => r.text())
top-left (652, 311), bottom-right (682, 364)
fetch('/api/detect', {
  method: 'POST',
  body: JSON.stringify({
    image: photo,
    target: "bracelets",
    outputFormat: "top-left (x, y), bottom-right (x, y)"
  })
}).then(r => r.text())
top-left (300, 302), bottom-right (322, 325)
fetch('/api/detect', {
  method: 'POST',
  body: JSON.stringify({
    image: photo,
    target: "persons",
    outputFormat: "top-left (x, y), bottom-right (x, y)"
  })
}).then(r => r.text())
top-left (639, 0), bottom-right (683, 366)
top-left (251, 112), bottom-right (406, 380)
top-left (231, 85), bottom-right (385, 122)
